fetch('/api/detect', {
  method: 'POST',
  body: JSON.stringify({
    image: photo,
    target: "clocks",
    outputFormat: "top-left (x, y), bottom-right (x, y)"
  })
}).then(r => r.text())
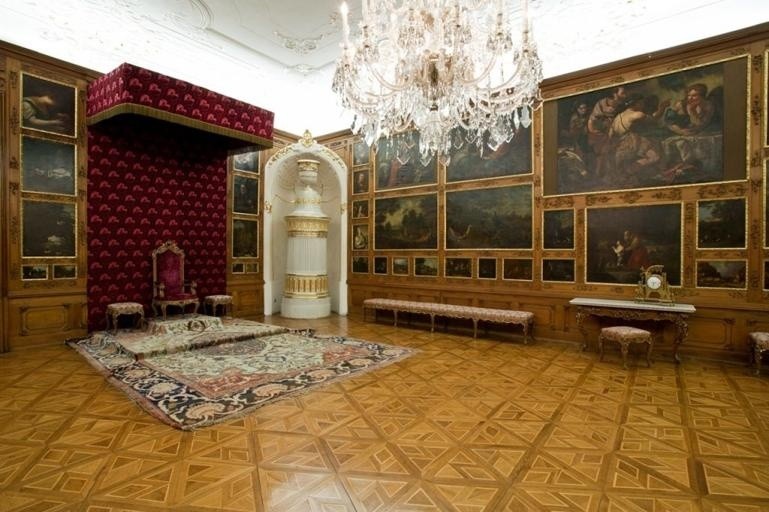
top-left (633, 264), bottom-right (677, 307)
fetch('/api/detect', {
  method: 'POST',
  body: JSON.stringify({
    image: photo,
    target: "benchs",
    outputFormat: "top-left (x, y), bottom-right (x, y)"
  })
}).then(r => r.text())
top-left (362, 296), bottom-right (538, 345)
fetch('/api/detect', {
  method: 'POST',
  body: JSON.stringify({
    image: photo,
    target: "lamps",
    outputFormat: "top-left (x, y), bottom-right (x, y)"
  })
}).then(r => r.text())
top-left (330, 0), bottom-right (546, 169)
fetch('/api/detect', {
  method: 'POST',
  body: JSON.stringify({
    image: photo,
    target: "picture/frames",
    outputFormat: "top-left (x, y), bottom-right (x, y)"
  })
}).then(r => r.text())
top-left (18, 70), bottom-right (80, 282)
top-left (230, 147), bottom-right (261, 276)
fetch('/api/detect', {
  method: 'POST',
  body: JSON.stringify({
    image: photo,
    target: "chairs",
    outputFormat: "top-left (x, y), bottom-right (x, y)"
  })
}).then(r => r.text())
top-left (150, 238), bottom-right (201, 321)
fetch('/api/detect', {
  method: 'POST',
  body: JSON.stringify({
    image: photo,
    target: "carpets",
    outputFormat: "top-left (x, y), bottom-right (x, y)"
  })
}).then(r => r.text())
top-left (63, 312), bottom-right (424, 433)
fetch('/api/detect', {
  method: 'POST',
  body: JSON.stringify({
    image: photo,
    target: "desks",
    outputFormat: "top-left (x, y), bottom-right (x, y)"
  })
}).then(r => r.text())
top-left (567, 295), bottom-right (698, 365)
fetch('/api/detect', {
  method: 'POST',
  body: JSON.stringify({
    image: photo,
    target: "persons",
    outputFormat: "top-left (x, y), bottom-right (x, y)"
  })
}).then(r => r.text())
top-left (612, 240), bottom-right (627, 269)
top-left (354, 227), bottom-right (368, 249)
top-left (20, 85), bottom-right (73, 129)
top-left (622, 229), bottom-right (650, 271)
top-left (555, 84), bottom-right (724, 191)
top-left (354, 203), bottom-right (367, 217)
top-left (234, 181), bottom-right (258, 214)
top-left (354, 173), bottom-right (370, 194)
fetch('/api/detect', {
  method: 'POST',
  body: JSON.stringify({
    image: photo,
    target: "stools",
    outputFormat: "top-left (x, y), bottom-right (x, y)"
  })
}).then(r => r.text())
top-left (202, 293), bottom-right (234, 317)
top-left (744, 330), bottom-right (769, 376)
top-left (104, 301), bottom-right (148, 336)
top-left (598, 324), bottom-right (655, 371)
